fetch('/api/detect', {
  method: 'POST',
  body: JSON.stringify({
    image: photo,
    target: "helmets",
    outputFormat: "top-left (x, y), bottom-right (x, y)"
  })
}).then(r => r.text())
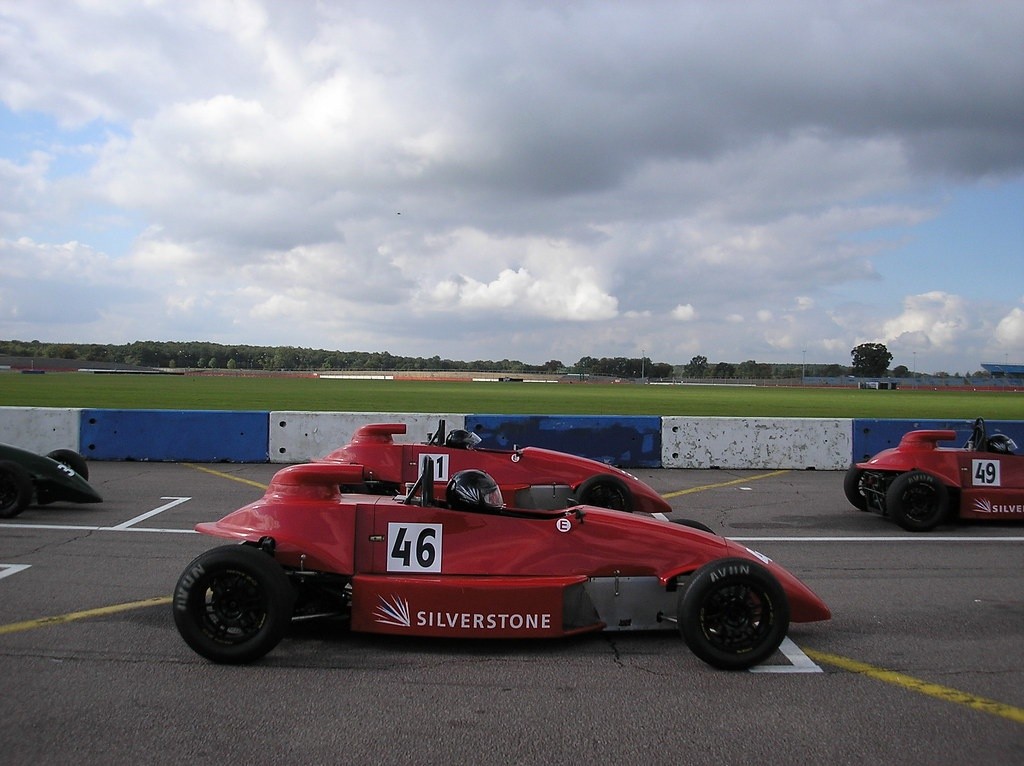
top-left (987, 434), bottom-right (1018, 453)
top-left (445, 430), bottom-right (481, 451)
top-left (445, 469), bottom-right (503, 509)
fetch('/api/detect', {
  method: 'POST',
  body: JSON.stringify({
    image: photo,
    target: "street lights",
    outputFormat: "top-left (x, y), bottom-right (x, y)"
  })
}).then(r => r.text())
top-left (641, 349), bottom-right (646, 379)
top-left (802, 350), bottom-right (807, 379)
top-left (913, 351), bottom-right (916, 380)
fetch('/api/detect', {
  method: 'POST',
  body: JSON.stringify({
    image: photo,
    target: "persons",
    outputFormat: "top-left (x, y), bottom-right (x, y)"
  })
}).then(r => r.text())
top-left (986, 434), bottom-right (1018, 455)
top-left (446, 429), bottom-right (482, 451)
top-left (445, 469), bottom-right (503, 510)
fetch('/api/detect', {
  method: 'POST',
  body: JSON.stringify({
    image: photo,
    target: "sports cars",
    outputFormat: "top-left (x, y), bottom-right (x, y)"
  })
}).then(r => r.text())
top-left (308, 419), bottom-right (675, 518)
top-left (173, 455), bottom-right (833, 673)
top-left (0, 442), bottom-right (103, 519)
top-left (843, 416), bottom-right (1024, 532)
